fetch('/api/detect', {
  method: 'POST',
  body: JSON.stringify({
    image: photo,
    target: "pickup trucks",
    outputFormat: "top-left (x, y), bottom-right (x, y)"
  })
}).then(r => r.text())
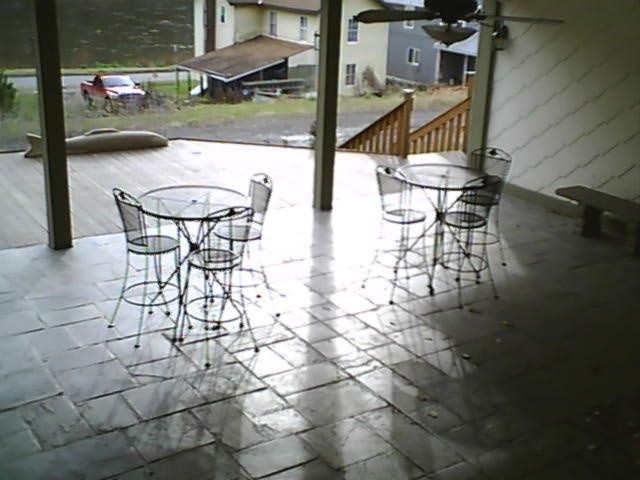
top-left (81, 73), bottom-right (147, 114)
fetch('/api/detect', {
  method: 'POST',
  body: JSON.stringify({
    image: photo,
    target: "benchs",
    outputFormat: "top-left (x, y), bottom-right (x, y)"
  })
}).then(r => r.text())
top-left (555, 185), bottom-right (640, 238)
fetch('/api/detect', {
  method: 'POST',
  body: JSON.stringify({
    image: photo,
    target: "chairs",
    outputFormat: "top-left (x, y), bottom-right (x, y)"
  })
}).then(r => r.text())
top-left (362, 145), bottom-right (513, 309)
top-left (108, 172), bottom-right (274, 368)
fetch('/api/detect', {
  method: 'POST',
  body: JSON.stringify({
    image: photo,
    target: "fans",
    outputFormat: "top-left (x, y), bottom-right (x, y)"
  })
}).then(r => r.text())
top-left (353, 0), bottom-right (567, 31)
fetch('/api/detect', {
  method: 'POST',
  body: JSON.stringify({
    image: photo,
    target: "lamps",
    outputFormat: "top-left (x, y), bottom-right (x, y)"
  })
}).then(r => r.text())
top-left (491, 24), bottom-right (507, 50)
top-left (420, 16), bottom-right (478, 48)
